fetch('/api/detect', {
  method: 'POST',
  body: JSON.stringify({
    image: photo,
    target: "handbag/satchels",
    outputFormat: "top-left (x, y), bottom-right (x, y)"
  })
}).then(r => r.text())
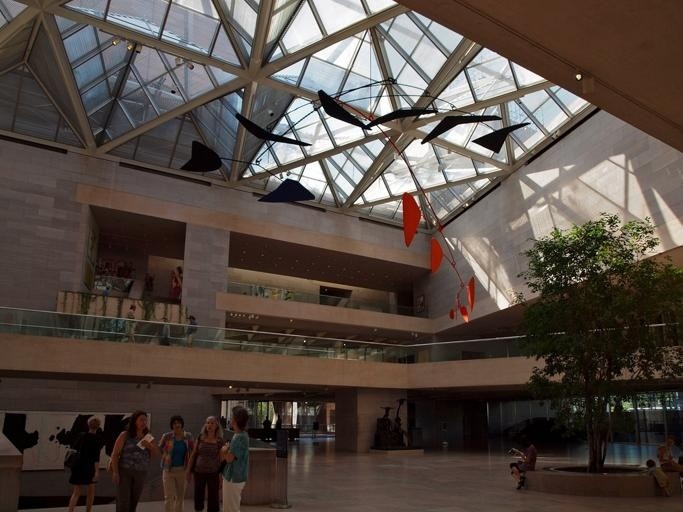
top-left (65, 450), bottom-right (77, 468)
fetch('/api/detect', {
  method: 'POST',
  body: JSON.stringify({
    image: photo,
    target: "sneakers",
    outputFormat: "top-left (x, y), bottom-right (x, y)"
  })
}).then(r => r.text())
top-left (516, 476), bottom-right (526, 489)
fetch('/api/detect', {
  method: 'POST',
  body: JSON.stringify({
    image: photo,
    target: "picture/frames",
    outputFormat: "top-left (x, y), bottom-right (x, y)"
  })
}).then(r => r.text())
top-left (0, 410), bottom-right (152, 472)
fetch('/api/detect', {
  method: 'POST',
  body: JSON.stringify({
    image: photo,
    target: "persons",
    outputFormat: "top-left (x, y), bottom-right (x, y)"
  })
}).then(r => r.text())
top-left (645, 459), bottom-right (672, 496)
top-left (510, 442), bottom-right (537, 489)
top-left (65, 414), bottom-right (104, 512)
top-left (263, 414), bottom-right (271, 440)
top-left (185, 416), bottom-right (224, 512)
top-left (276, 412), bottom-right (283, 430)
top-left (158, 415), bottom-right (194, 512)
top-left (219, 405), bottom-right (251, 512)
top-left (110, 411), bottom-right (155, 512)
top-left (95, 253), bottom-right (199, 346)
top-left (659, 437), bottom-right (683, 484)
